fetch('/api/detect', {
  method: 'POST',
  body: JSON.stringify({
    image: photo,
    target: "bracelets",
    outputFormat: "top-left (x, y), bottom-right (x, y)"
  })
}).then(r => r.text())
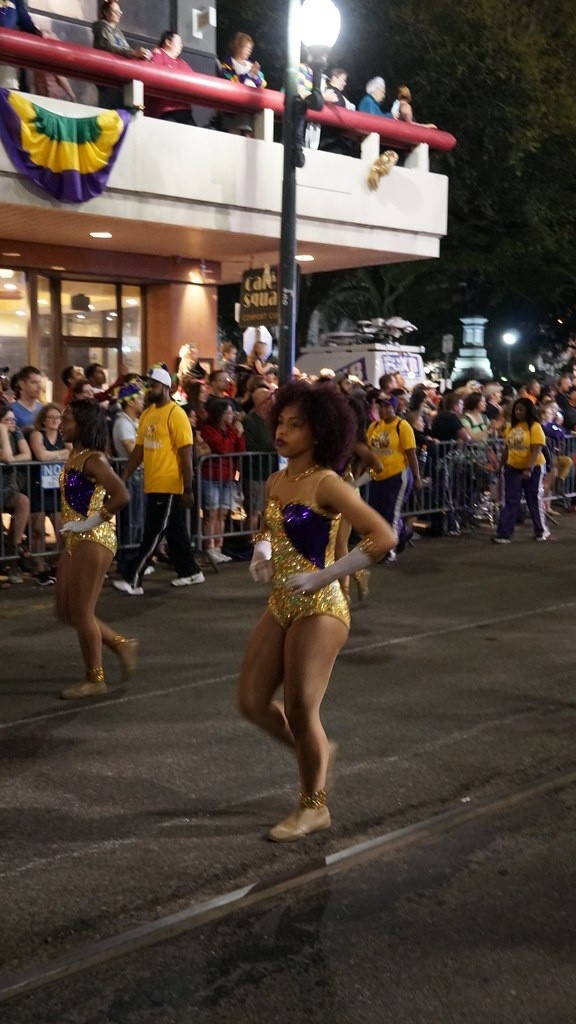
top-left (527, 467), bottom-right (533, 474)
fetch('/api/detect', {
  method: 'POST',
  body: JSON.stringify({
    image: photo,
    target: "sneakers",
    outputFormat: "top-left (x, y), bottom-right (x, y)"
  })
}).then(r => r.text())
top-left (171, 570), bottom-right (206, 586)
top-left (112, 580), bottom-right (143, 595)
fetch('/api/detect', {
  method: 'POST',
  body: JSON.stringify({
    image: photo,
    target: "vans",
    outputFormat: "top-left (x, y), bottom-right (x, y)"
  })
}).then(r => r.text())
top-left (294, 345), bottom-right (424, 391)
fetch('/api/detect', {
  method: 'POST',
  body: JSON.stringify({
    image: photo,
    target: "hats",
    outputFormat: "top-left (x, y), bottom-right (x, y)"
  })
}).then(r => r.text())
top-left (137, 362), bottom-right (172, 389)
top-left (483, 384), bottom-right (504, 397)
top-left (421, 380), bottom-right (440, 389)
top-left (466, 380), bottom-right (484, 390)
top-left (0, 366), bottom-right (9, 373)
top-left (375, 393), bottom-right (398, 410)
top-left (117, 384), bottom-right (144, 404)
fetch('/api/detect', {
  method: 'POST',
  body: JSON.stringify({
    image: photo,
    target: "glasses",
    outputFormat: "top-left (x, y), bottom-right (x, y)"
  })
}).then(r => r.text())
top-left (45, 414), bottom-right (63, 421)
top-left (216, 377), bottom-right (231, 383)
top-left (223, 410), bottom-right (235, 417)
top-left (4, 417), bottom-right (18, 423)
top-left (0, 375), bottom-right (10, 381)
top-left (256, 393), bottom-right (273, 407)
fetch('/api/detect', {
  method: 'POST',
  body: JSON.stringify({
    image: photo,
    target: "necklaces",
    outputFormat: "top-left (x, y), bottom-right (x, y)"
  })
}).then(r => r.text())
top-left (285, 463), bottom-right (320, 481)
top-left (69, 448), bottom-right (91, 459)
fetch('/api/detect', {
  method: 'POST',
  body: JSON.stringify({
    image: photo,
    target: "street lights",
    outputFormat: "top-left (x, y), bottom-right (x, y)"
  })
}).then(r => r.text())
top-left (276, 0), bottom-right (342, 378)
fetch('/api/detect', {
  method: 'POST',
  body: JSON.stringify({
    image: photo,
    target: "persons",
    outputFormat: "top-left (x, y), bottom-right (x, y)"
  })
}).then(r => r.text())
top-left (0, 0), bottom-right (50, 91)
top-left (145, 30), bottom-right (197, 127)
top-left (280, 46), bottom-right (356, 156)
top-left (491, 398), bottom-right (550, 542)
top-left (222, 33), bottom-right (267, 138)
top-left (0, 363), bottom-right (172, 585)
top-left (170, 342), bottom-right (281, 565)
top-left (237, 382), bottom-right (396, 840)
top-left (296, 369), bottom-right (576, 539)
top-left (93, 0), bottom-right (152, 109)
top-left (360, 77), bottom-right (437, 166)
top-left (355, 395), bottom-right (422, 560)
top-left (336, 400), bottom-right (384, 604)
top-left (56, 400), bottom-right (139, 698)
top-left (111, 367), bottom-right (205, 593)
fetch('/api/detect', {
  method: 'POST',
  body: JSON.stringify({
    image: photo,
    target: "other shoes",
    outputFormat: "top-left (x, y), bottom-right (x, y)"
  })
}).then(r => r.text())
top-left (394, 530), bottom-right (413, 553)
top-left (268, 808), bottom-right (332, 842)
top-left (59, 679), bottom-right (110, 701)
top-left (31, 569), bottom-right (56, 587)
top-left (534, 529), bottom-right (551, 541)
top-left (117, 638), bottom-right (137, 678)
top-left (492, 535), bottom-right (512, 544)
top-left (205, 546), bottom-right (232, 564)
